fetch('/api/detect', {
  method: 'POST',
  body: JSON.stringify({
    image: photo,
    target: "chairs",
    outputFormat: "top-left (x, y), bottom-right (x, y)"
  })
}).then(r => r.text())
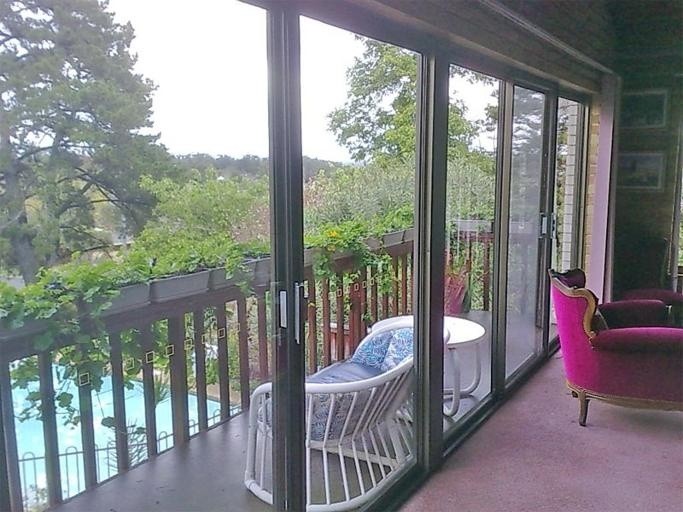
top-left (614, 231), bottom-right (683, 325)
top-left (243, 320), bottom-right (451, 511)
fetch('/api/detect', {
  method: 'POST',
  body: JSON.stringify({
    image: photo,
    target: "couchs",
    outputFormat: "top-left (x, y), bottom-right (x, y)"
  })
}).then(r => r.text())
top-left (544, 265), bottom-right (683, 427)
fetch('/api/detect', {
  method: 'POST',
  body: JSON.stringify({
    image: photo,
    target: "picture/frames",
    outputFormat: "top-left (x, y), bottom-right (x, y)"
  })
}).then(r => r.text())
top-left (616, 147), bottom-right (669, 195)
top-left (617, 87), bottom-right (674, 134)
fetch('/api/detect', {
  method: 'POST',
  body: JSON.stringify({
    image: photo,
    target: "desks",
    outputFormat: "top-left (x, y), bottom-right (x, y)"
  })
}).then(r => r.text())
top-left (369, 309), bottom-right (486, 424)
